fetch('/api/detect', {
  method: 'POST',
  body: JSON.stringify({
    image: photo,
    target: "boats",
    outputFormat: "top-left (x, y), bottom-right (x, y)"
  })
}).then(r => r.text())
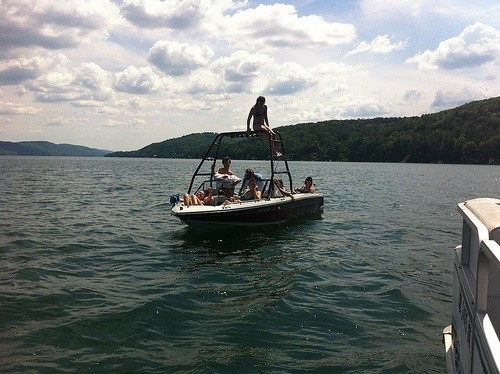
top-left (442, 197), bottom-right (500, 374)
top-left (171, 130), bottom-right (324, 223)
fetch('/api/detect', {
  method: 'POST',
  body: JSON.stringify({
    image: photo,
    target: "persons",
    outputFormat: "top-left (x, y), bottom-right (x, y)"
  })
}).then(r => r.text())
top-left (230, 179), bottom-right (261, 201)
top-left (179, 187), bottom-right (212, 206)
top-left (247, 96), bottom-right (282, 157)
top-left (243, 168), bottom-right (269, 193)
top-left (265, 178), bottom-right (286, 197)
top-left (215, 157), bottom-right (241, 197)
top-left (294, 177), bottom-right (315, 194)
